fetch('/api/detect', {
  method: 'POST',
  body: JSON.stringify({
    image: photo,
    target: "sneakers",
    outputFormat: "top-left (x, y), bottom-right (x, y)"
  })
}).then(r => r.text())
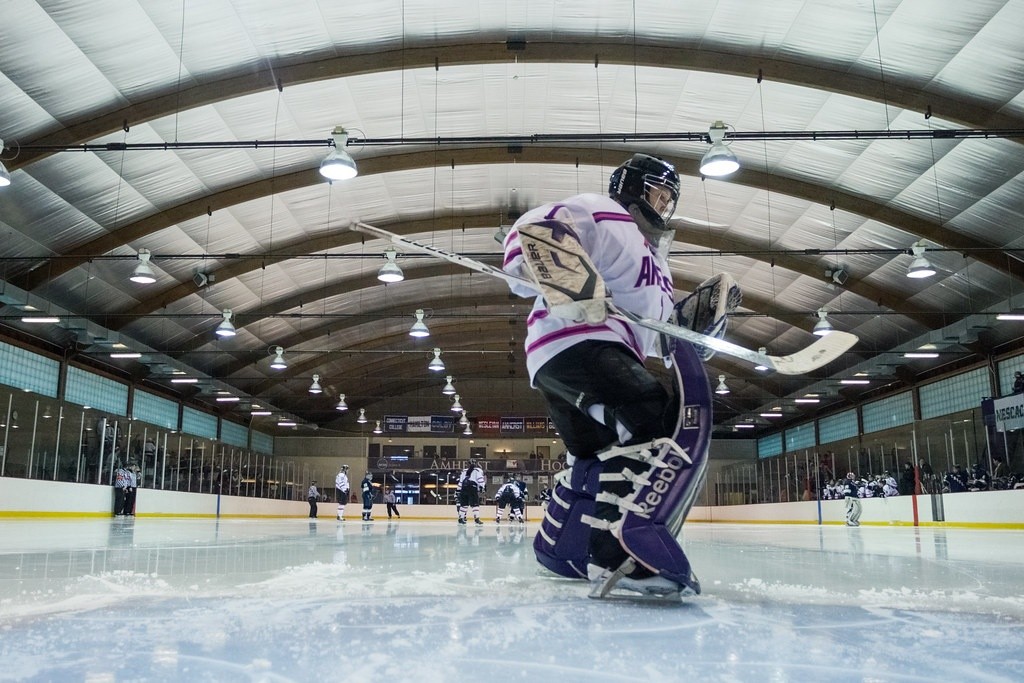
top-left (509, 516), bottom-right (514, 524)
top-left (496, 516), bottom-right (500, 524)
top-left (362, 515), bottom-right (374, 522)
top-left (337, 515), bottom-right (345, 522)
top-left (588, 530), bottom-right (683, 603)
top-left (457, 517), bottom-right (467, 526)
top-left (535, 556), bottom-right (589, 583)
top-left (474, 517), bottom-right (483, 526)
top-left (519, 517), bottom-right (525, 525)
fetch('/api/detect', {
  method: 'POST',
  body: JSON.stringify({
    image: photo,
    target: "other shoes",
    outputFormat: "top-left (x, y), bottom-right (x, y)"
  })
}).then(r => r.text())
top-left (116, 513), bottom-right (134, 518)
top-left (309, 515), bottom-right (317, 519)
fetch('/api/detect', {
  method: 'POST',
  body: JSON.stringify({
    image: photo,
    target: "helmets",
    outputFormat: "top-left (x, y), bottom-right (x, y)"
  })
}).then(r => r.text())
top-left (507, 477), bottom-right (514, 484)
top-left (827, 469), bottom-right (891, 484)
top-left (312, 480), bottom-right (317, 486)
top-left (847, 472), bottom-right (856, 480)
top-left (341, 464), bottom-right (350, 471)
top-left (467, 458), bottom-right (476, 467)
top-left (609, 153), bottom-right (681, 248)
top-left (364, 471), bottom-right (372, 480)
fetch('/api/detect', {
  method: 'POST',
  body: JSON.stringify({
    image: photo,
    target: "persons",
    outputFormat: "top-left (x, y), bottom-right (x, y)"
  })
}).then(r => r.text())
top-left (335, 464), bottom-right (350, 521)
top-left (503, 153), bottom-right (743, 604)
top-left (747, 371), bottom-right (1024, 526)
top-left (308, 481), bottom-right (321, 518)
top-left (424, 451), bottom-right (568, 525)
top-left (361, 472), bottom-right (375, 521)
top-left (90, 418), bottom-right (272, 499)
top-left (114, 461), bottom-right (142, 516)
top-left (384, 486), bottom-right (400, 519)
top-left (320, 491), bottom-right (358, 503)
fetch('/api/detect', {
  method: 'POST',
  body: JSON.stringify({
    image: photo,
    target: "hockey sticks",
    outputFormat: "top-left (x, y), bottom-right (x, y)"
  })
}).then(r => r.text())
top-left (347, 216), bottom-right (860, 377)
